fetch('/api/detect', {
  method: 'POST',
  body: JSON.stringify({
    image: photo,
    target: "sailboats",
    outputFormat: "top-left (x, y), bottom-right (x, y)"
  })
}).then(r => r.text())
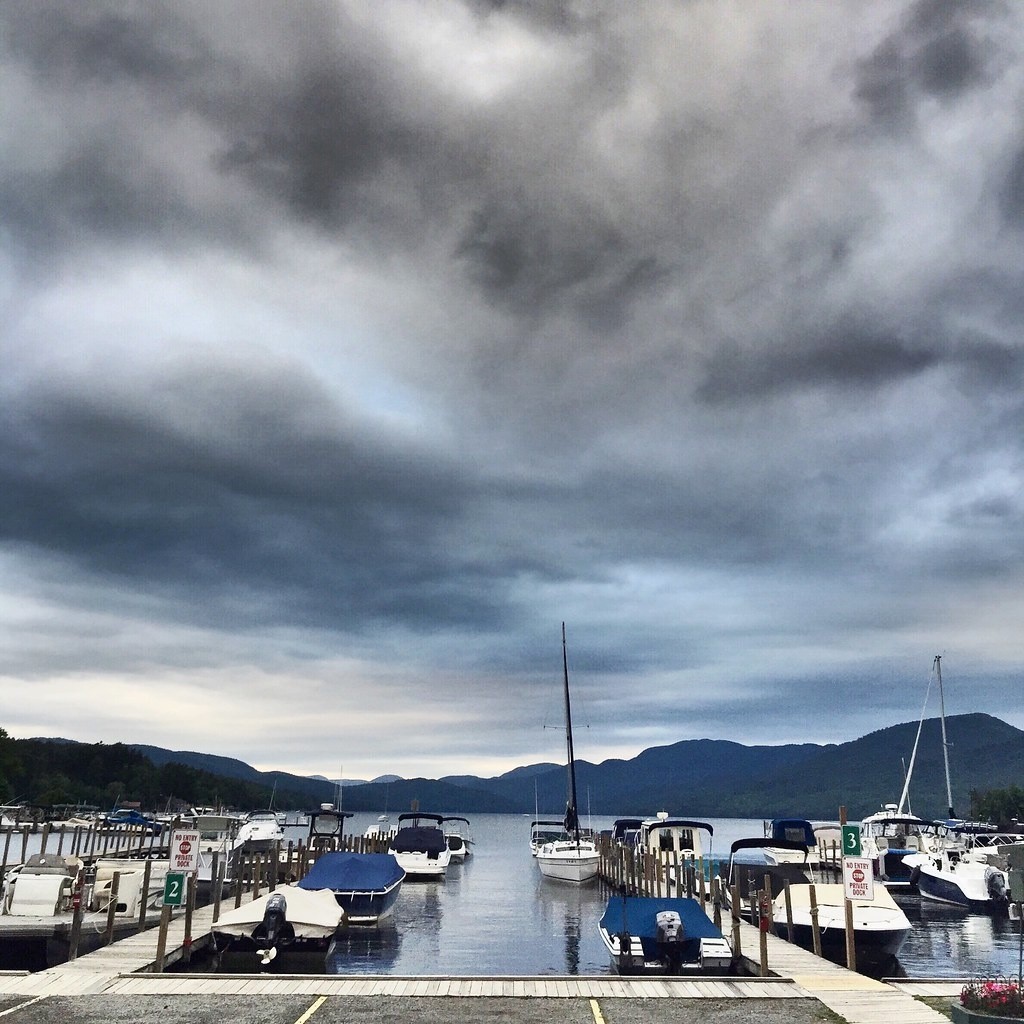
top-left (263, 778), bottom-right (285, 819)
top-left (858, 653), bottom-right (1023, 886)
top-left (535, 618), bottom-right (601, 884)
top-left (378, 778), bottom-right (389, 823)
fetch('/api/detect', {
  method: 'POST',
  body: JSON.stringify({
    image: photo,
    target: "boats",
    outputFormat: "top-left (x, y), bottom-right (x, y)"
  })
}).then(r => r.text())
top-left (600, 894), bottom-right (733, 976)
top-left (1, 857), bottom-right (195, 966)
top-left (5, 808), bottom-right (247, 835)
top-left (213, 882), bottom-right (348, 964)
top-left (298, 849), bottom-right (406, 922)
top-left (530, 820), bottom-right (570, 856)
top-left (437, 817), bottom-right (478, 863)
top-left (760, 816), bottom-right (815, 871)
top-left (229, 809), bottom-right (309, 884)
top-left (174, 803), bottom-right (253, 896)
top-left (720, 838), bottom-right (818, 922)
top-left (764, 882), bottom-right (910, 975)
top-left (903, 818), bottom-right (1024, 908)
top-left (388, 813), bottom-right (446, 880)
top-left (601, 808), bottom-right (718, 900)
